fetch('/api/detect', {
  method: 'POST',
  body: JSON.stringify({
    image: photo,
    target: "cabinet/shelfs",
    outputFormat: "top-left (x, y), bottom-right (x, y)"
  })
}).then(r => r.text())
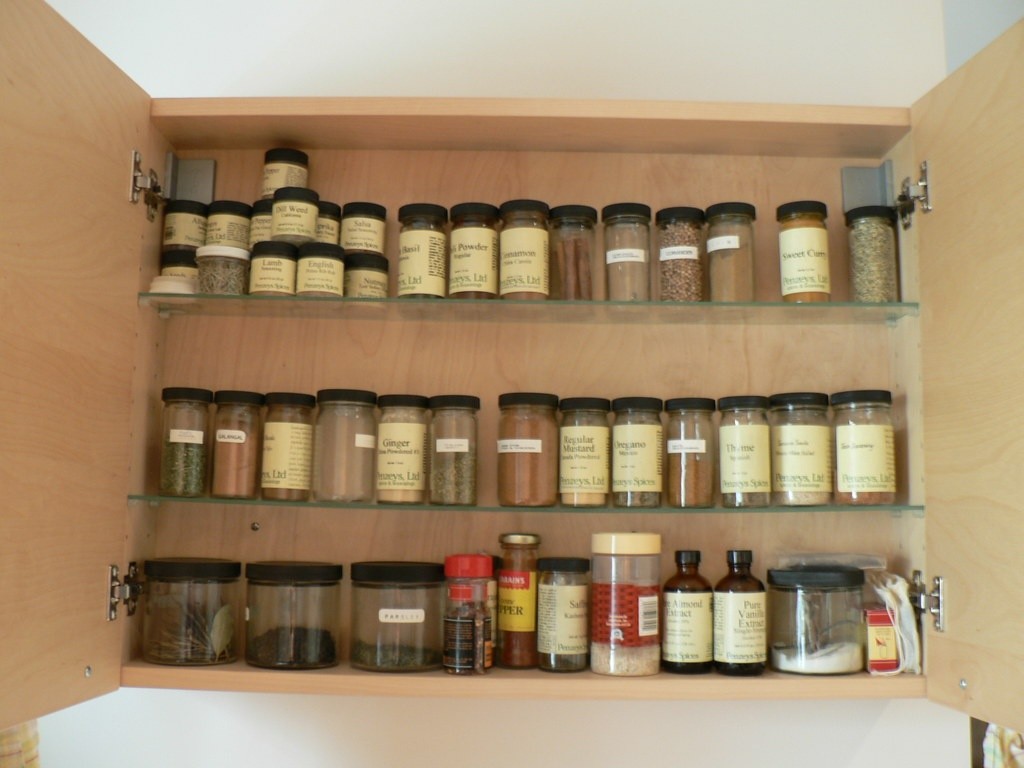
top-left (0, 0), bottom-right (1023, 740)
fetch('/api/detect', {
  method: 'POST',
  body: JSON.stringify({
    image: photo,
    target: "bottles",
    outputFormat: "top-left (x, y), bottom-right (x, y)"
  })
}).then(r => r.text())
top-left (143, 554), bottom-right (497, 675)
top-left (160, 148), bottom-right (388, 297)
top-left (159, 387), bottom-right (897, 507)
top-left (497, 532), bottom-right (864, 676)
top-left (397, 200), bottom-right (898, 301)
